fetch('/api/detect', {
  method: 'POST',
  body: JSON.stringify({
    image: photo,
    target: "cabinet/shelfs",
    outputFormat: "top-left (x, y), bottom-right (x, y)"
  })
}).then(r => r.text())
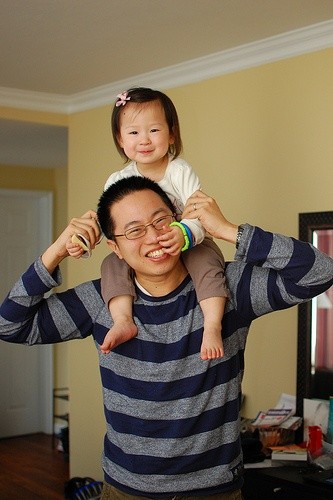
top-left (52, 388), bottom-right (69, 453)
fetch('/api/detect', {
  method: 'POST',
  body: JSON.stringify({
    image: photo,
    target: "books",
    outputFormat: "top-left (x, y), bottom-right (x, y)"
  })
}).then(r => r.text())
top-left (272, 448), bottom-right (308, 460)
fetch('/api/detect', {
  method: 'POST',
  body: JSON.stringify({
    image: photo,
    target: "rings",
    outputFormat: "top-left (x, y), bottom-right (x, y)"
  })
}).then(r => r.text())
top-left (193, 203), bottom-right (197, 210)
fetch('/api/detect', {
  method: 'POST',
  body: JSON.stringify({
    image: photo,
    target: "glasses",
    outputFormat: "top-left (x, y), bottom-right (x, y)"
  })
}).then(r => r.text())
top-left (112, 215), bottom-right (176, 240)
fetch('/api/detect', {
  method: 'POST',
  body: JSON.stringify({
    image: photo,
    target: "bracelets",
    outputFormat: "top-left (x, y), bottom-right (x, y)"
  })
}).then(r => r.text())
top-left (180, 223), bottom-right (193, 250)
top-left (169, 221), bottom-right (190, 251)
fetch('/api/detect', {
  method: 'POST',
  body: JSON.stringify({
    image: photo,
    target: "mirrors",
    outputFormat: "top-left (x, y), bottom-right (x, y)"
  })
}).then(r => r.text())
top-left (297, 211), bottom-right (333, 445)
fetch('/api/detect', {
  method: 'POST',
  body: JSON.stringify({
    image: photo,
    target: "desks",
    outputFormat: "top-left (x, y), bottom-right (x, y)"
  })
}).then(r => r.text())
top-left (240, 458), bottom-right (333, 500)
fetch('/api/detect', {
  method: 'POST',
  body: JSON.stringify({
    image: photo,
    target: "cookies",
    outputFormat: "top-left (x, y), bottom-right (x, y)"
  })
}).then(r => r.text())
top-left (71, 235), bottom-right (89, 250)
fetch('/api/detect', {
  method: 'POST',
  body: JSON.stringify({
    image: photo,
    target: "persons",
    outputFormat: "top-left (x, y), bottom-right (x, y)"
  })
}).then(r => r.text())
top-left (65, 87), bottom-right (233, 361)
top-left (0, 175), bottom-right (333, 500)
